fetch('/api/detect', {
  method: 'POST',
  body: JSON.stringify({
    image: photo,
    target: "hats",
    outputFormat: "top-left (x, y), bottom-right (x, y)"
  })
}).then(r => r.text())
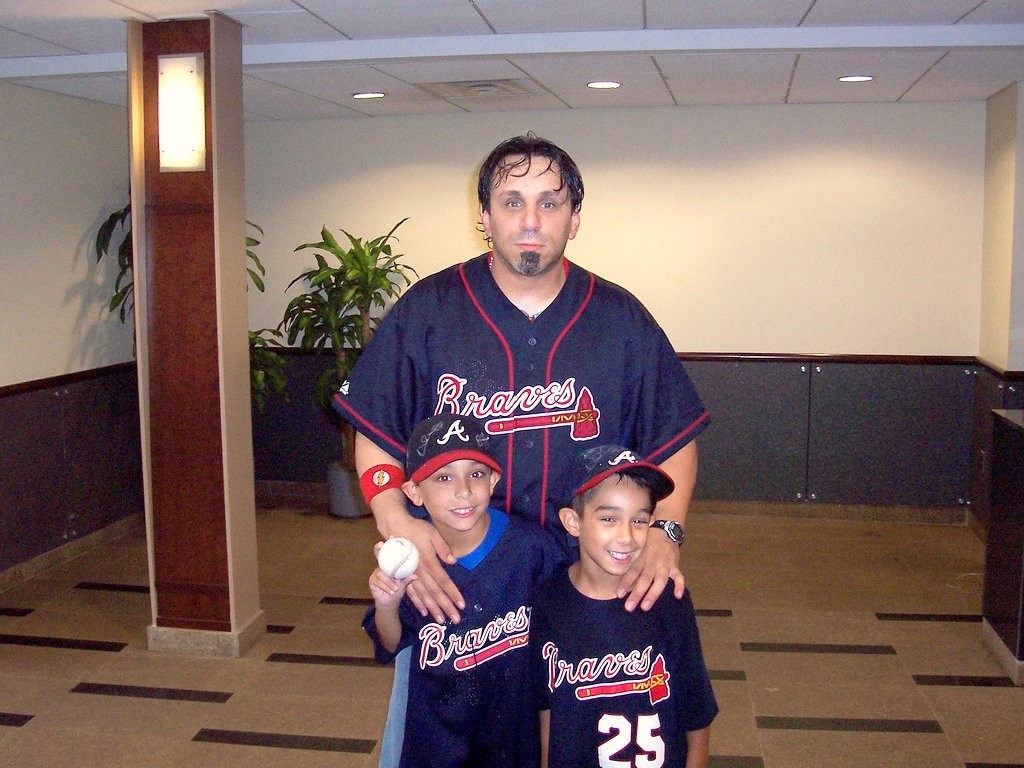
top-left (406, 415), bottom-right (505, 483)
top-left (565, 445), bottom-right (675, 507)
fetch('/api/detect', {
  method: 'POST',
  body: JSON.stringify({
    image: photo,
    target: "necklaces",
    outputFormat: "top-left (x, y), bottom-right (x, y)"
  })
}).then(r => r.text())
top-left (485, 250), bottom-right (543, 321)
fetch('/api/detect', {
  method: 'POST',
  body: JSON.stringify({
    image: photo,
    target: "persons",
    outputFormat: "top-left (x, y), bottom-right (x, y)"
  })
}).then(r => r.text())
top-left (358, 414), bottom-right (571, 768)
top-left (525, 445), bottom-right (718, 768)
top-left (328, 130), bottom-right (709, 768)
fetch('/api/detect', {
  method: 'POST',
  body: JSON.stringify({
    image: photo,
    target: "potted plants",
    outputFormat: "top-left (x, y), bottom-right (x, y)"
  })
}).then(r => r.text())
top-left (277, 217), bottom-right (419, 518)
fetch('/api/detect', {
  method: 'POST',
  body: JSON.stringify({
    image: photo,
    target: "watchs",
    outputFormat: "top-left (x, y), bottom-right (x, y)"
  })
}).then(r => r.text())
top-left (650, 519), bottom-right (686, 546)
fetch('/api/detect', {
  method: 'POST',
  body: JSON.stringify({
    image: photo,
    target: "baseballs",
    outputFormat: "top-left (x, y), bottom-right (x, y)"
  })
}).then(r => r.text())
top-left (378, 538), bottom-right (420, 578)
